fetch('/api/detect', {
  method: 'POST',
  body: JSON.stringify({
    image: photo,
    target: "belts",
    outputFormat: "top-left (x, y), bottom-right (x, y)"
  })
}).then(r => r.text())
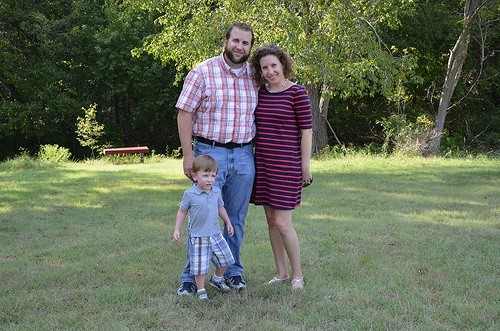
top-left (193, 136), bottom-right (256, 149)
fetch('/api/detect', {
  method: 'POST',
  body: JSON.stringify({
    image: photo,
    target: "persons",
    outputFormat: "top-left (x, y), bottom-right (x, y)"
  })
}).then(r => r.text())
top-left (176, 22), bottom-right (261, 296)
top-left (173, 154), bottom-right (236, 301)
top-left (249, 44), bottom-right (313, 290)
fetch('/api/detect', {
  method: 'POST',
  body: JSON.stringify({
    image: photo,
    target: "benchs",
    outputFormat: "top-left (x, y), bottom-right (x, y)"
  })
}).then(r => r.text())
top-left (104, 146), bottom-right (149, 165)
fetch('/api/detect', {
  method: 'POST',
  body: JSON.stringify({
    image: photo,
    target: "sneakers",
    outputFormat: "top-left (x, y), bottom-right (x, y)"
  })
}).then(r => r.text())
top-left (225, 275), bottom-right (247, 291)
top-left (268, 275), bottom-right (289, 285)
top-left (209, 274), bottom-right (231, 293)
top-left (302, 175), bottom-right (313, 187)
top-left (197, 290), bottom-right (208, 300)
top-left (177, 282), bottom-right (197, 296)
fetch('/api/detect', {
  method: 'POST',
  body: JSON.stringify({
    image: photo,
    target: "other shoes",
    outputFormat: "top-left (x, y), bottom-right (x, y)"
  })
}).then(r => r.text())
top-left (291, 275), bottom-right (305, 289)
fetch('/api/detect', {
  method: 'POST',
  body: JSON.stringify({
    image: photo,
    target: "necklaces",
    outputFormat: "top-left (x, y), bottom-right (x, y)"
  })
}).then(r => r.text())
top-left (265, 78), bottom-right (288, 93)
